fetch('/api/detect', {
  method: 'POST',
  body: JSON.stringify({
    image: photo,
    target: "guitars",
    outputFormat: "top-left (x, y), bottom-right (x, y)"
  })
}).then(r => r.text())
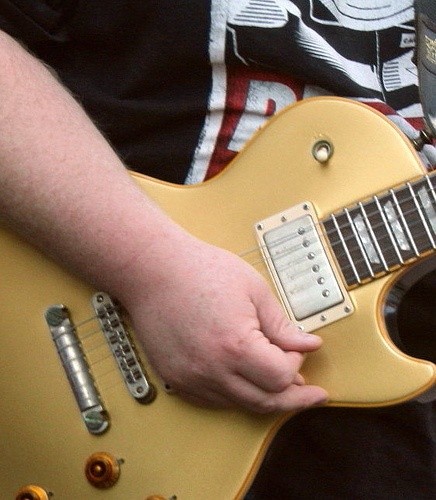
top-left (1, 95), bottom-right (434, 499)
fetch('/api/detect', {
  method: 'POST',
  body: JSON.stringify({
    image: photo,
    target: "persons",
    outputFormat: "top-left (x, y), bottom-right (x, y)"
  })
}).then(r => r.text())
top-left (0, 1), bottom-right (435, 499)
